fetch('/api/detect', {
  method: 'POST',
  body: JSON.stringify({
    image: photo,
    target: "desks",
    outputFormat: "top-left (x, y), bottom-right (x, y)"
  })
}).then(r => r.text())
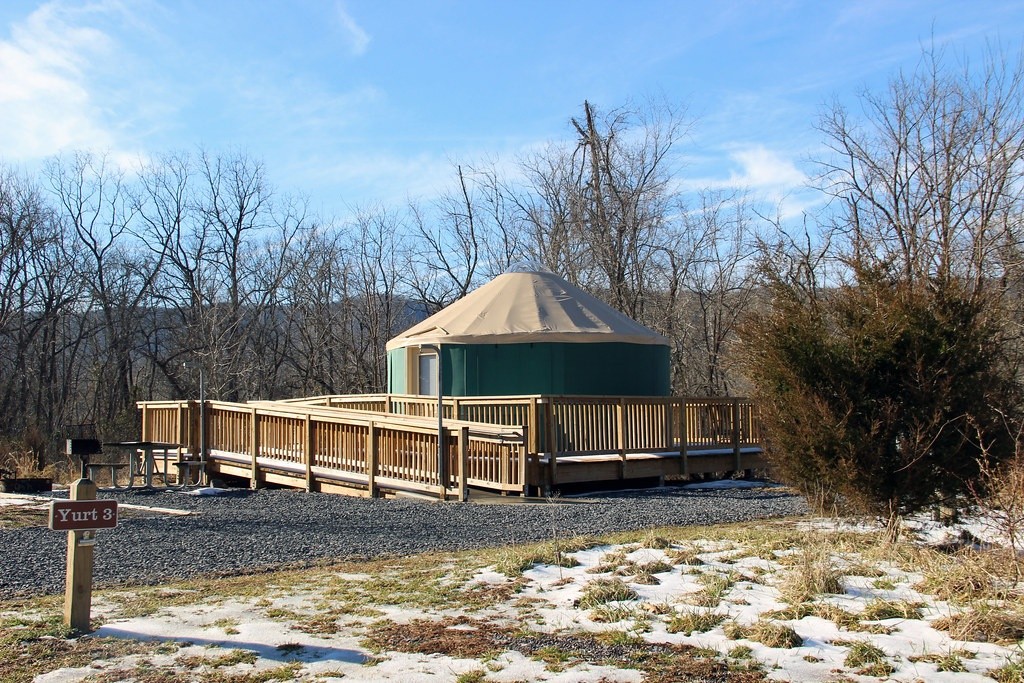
top-left (104, 441), bottom-right (183, 490)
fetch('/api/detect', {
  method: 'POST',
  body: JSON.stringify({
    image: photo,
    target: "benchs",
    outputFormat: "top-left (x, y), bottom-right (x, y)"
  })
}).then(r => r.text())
top-left (85, 463), bottom-right (131, 492)
top-left (171, 461), bottom-right (207, 491)
